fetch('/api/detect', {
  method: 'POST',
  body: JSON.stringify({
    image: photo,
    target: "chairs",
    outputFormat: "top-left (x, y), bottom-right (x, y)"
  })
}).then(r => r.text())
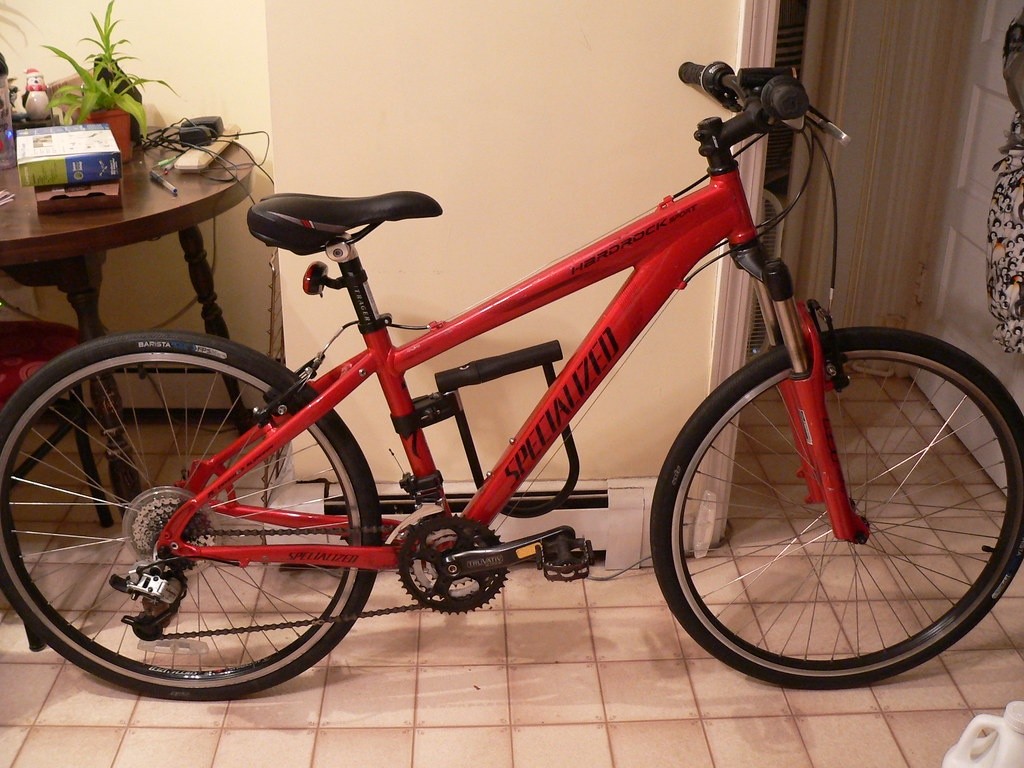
top-left (0, 321), bottom-right (114, 653)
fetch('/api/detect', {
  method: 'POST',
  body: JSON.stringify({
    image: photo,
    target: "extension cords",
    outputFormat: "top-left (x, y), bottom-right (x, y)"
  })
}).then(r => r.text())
top-left (175, 125), bottom-right (241, 173)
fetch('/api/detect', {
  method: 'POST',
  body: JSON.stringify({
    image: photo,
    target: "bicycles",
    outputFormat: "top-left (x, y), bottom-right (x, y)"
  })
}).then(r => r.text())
top-left (0, 58), bottom-right (1024, 705)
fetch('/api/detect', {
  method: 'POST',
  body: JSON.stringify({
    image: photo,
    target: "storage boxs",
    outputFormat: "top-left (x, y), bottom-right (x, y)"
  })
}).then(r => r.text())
top-left (17, 123), bottom-right (123, 186)
top-left (0, 74), bottom-right (16, 169)
top-left (34, 179), bottom-right (123, 213)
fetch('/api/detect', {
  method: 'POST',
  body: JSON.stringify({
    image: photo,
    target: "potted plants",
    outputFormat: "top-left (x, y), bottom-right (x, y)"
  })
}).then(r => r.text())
top-left (39, 0), bottom-right (181, 164)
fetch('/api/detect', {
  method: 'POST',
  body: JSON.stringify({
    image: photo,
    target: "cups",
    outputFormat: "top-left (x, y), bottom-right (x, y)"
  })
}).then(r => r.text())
top-left (24, 91), bottom-right (50, 120)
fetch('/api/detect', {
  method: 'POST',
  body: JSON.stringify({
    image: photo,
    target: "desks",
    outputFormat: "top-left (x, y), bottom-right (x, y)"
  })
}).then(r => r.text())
top-left (0, 115), bottom-right (254, 520)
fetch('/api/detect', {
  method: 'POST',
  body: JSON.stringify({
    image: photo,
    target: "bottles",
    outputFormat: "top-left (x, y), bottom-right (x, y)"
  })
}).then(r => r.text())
top-left (941, 700), bottom-right (1024, 768)
top-left (0, 52), bottom-right (16, 171)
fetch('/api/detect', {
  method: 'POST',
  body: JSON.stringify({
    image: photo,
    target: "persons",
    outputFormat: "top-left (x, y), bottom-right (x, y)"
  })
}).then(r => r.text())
top-left (984, 8), bottom-right (1023, 355)
